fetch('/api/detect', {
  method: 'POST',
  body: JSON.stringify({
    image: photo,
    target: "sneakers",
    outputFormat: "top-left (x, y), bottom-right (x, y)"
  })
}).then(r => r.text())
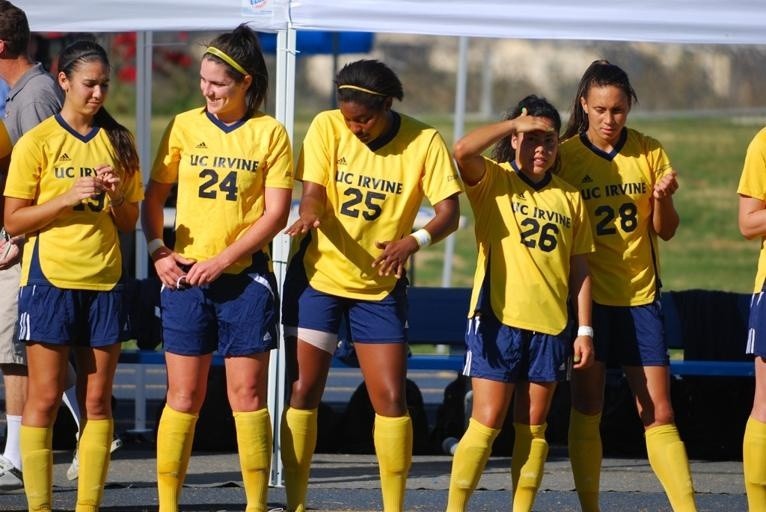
top-left (65, 430), bottom-right (122, 480)
top-left (0, 456), bottom-right (25, 494)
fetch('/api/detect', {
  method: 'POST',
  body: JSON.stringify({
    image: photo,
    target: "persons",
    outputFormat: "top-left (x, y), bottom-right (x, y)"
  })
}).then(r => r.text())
top-left (141, 21), bottom-right (295, 512)
top-left (444, 94), bottom-right (598, 512)
top-left (550, 58), bottom-right (698, 512)
top-left (278, 59), bottom-right (465, 512)
top-left (4, 32), bottom-right (145, 511)
top-left (0, 0), bottom-right (124, 490)
top-left (735, 126), bottom-right (766, 512)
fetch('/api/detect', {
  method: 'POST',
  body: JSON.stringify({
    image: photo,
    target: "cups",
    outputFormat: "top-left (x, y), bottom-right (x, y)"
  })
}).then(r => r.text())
top-left (442, 437), bottom-right (459, 455)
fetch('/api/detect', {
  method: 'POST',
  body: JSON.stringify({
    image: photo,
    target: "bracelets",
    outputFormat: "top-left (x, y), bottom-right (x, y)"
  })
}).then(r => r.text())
top-left (576, 325), bottom-right (594, 340)
top-left (409, 228), bottom-right (433, 251)
top-left (145, 237), bottom-right (165, 256)
